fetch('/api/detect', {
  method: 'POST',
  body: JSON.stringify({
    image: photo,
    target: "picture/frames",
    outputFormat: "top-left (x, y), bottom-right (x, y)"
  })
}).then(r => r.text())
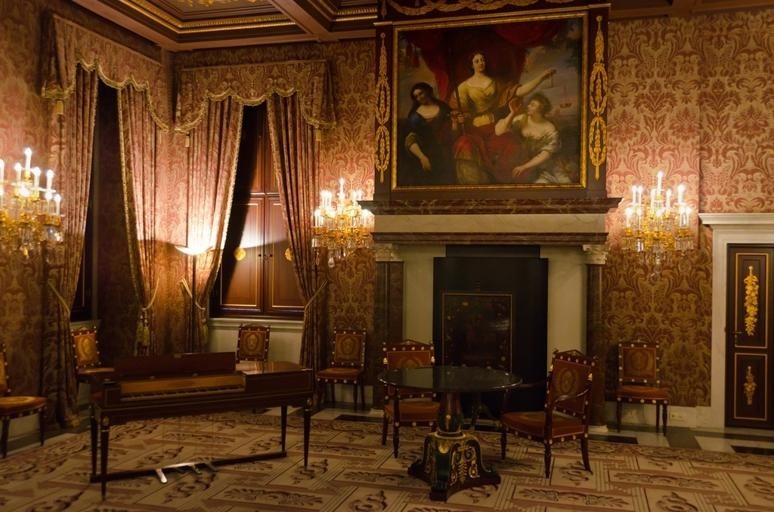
top-left (373, 5), bottom-right (608, 202)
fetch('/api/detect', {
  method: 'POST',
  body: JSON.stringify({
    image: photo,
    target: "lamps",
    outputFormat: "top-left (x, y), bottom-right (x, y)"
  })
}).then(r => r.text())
top-left (309, 177), bottom-right (375, 270)
top-left (622, 171), bottom-right (697, 269)
top-left (173, 214), bottom-right (215, 350)
top-left (0, 147), bottom-right (65, 259)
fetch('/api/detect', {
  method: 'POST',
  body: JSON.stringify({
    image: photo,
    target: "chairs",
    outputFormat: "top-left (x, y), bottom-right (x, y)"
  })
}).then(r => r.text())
top-left (68, 327), bottom-right (116, 417)
top-left (376, 337), bottom-right (447, 457)
top-left (615, 340), bottom-right (671, 436)
top-left (233, 321), bottom-right (270, 362)
top-left (315, 327), bottom-right (368, 412)
top-left (501, 349), bottom-right (597, 478)
top-left (0, 344), bottom-right (48, 458)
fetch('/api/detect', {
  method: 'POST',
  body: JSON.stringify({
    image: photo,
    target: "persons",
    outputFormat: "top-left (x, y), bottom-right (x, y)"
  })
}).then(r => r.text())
top-left (495, 93), bottom-right (572, 184)
top-left (449, 51), bottom-right (551, 184)
top-left (402, 82), bottom-right (452, 186)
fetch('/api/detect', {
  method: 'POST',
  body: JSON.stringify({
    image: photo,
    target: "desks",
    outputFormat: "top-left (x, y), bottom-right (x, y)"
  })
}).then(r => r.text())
top-left (375, 366), bottom-right (521, 502)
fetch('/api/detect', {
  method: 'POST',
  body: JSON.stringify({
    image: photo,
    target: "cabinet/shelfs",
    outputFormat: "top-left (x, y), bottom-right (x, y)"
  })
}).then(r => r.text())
top-left (215, 105), bottom-right (312, 316)
top-left (695, 212), bottom-right (774, 433)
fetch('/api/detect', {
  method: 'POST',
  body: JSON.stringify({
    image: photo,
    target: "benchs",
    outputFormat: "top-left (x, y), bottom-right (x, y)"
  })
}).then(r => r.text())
top-left (97, 350), bottom-right (249, 403)
